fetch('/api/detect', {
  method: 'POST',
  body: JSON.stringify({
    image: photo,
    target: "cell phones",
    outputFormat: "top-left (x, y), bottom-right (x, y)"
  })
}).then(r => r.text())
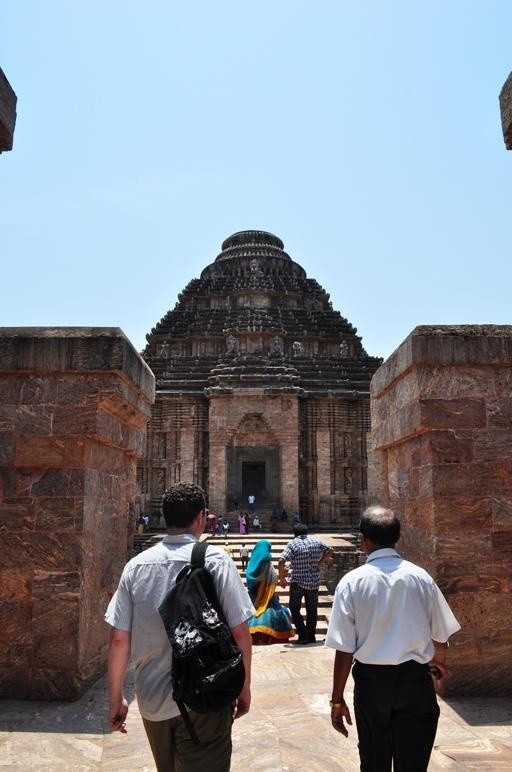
top-left (429, 666), bottom-right (442, 680)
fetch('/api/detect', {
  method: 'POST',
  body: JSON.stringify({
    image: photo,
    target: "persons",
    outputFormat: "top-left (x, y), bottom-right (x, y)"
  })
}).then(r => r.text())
top-left (136, 493), bottom-right (301, 572)
top-left (278, 523), bottom-right (334, 644)
top-left (246, 539), bottom-right (295, 644)
top-left (331, 505), bottom-right (462, 772)
top-left (104, 481), bottom-right (257, 772)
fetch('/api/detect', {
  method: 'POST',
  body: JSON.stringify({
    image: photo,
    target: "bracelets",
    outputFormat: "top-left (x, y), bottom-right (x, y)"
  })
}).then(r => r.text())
top-left (329, 700), bottom-right (345, 709)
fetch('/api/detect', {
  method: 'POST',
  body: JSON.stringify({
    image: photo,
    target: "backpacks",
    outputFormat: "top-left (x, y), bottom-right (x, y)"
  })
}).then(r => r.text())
top-left (157, 541), bottom-right (245, 713)
